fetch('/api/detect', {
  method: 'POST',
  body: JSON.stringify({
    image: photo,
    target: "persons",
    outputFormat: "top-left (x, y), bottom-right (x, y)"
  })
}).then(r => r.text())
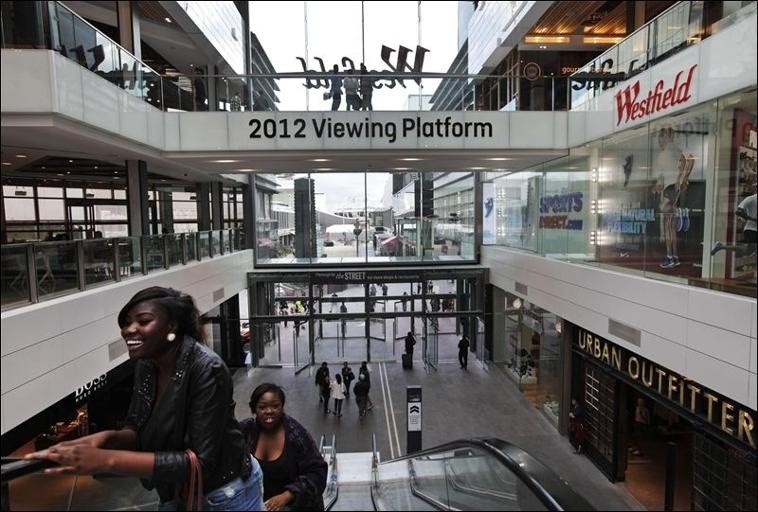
top-left (193, 72), bottom-right (205, 112)
top-left (458, 336), bottom-right (469, 371)
top-left (710, 194), bottom-right (757, 256)
top-left (369, 281), bottom-right (388, 312)
top-left (360, 66), bottom-right (383, 111)
top-left (315, 362), bottom-right (374, 420)
top-left (253, 91), bottom-right (269, 111)
top-left (340, 301), bottom-right (347, 325)
top-left (229, 93), bottom-right (241, 112)
top-left (237, 383), bottom-right (329, 512)
top-left (329, 292), bottom-right (338, 313)
top-left (279, 293), bottom-right (307, 328)
top-left (652, 131), bottom-right (696, 270)
top-left (418, 280), bottom-right (455, 314)
top-left (405, 332), bottom-right (416, 360)
top-left (87, 231), bottom-right (114, 276)
top-left (330, 65), bottom-right (342, 112)
top-left (22, 287), bottom-right (264, 512)
top-left (344, 69), bottom-right (359, 111)
top-left (401, 292), bottom-right (408, 312)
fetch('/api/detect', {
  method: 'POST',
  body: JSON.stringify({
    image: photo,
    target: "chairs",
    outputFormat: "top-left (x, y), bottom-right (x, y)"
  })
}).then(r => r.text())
top-left (11, 254), bottom-right (54, 289)
